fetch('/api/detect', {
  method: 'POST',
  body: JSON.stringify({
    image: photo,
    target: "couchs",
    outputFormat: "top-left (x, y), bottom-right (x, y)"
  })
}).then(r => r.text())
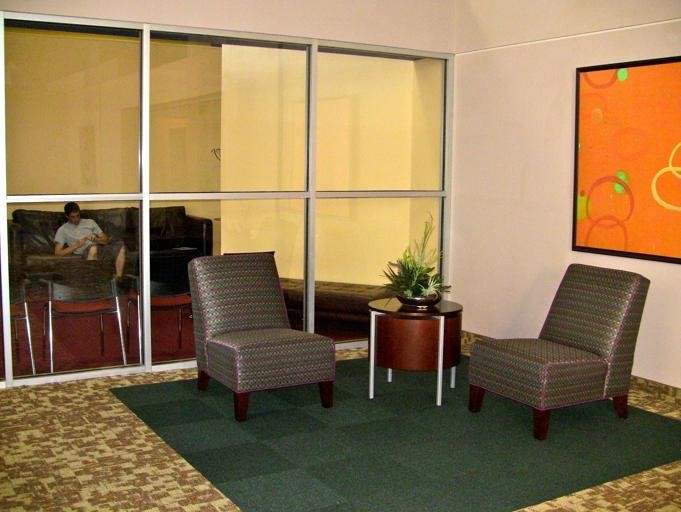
top-left (7, 215), bottom-right (213, 298)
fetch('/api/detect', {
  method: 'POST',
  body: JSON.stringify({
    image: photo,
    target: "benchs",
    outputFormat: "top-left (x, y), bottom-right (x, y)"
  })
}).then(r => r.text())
top-left (279, 277), bottom-right (398, 326)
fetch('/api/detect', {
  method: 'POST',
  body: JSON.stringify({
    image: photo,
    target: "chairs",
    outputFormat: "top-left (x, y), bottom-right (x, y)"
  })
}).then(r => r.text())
top-left (468, 263), bottom-right (651, 440)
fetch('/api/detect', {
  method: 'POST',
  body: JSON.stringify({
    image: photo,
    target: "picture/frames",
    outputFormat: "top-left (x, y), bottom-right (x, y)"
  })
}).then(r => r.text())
top-left (572, 55), bottom-right (681, 265)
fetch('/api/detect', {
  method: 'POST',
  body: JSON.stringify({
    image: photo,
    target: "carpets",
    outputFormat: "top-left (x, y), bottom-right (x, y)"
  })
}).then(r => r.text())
top-left (109, 354), bottom-right (681, 510)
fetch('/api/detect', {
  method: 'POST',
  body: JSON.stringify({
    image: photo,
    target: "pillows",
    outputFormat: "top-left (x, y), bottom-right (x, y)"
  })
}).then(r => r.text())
top-left (12, 206), bottom-right (187, 255)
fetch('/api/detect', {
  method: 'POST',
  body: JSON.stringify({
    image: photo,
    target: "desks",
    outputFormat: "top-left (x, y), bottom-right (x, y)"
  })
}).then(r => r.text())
top-left (366, 296), bottom-right (464, 406)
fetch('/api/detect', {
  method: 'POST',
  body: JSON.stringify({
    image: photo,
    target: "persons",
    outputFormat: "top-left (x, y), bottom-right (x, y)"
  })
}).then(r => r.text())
top-left (53, 202), bottom-right (126, 279)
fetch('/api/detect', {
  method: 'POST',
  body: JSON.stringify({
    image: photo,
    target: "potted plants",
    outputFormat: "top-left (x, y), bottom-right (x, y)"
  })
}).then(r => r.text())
top-left (378, 210), bottom-right (453, 309)
